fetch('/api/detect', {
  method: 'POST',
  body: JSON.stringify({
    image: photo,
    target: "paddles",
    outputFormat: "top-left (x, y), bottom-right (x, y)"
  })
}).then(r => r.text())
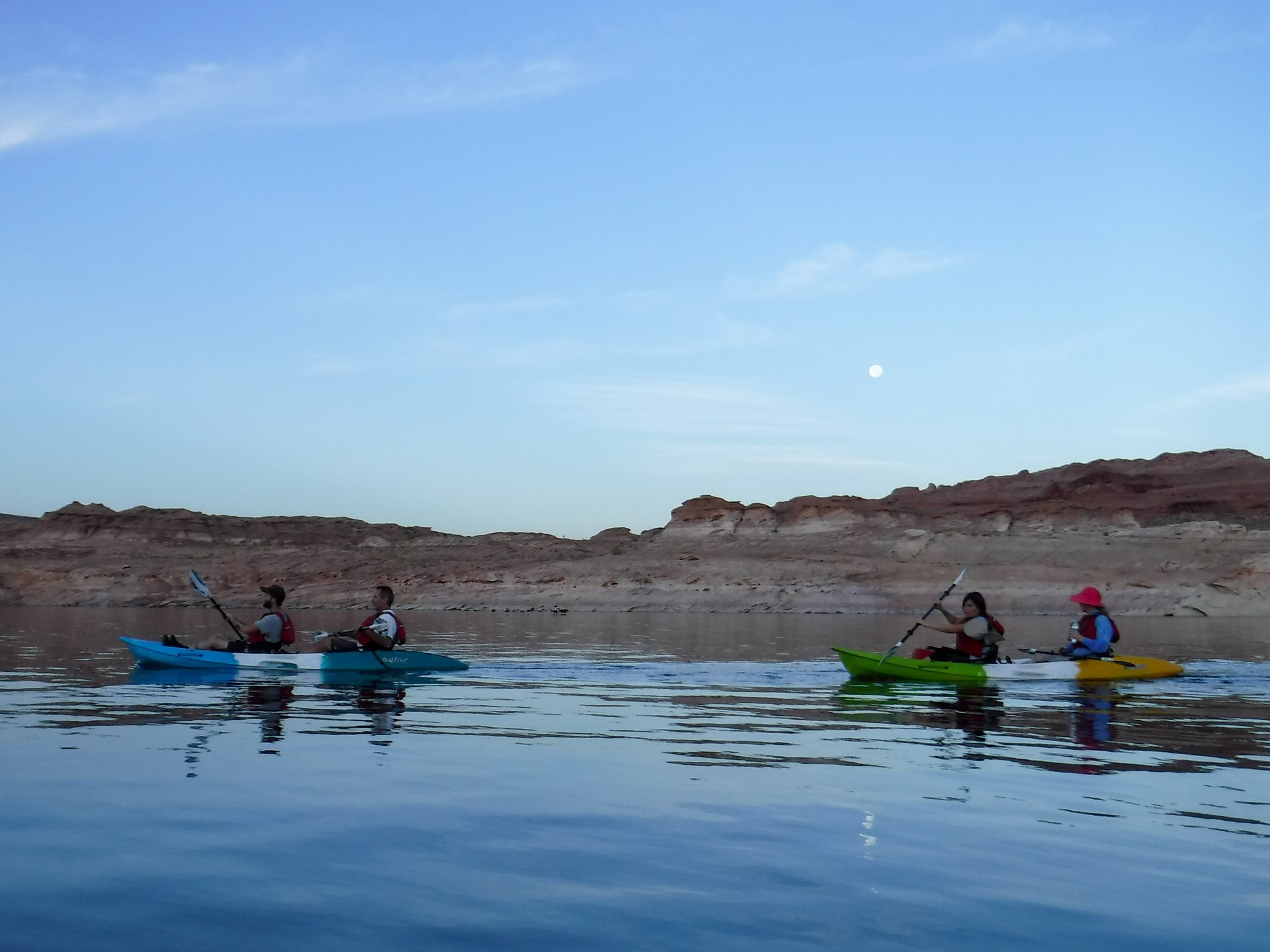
top-left (311, 617), bottom-right (391, 643)
top-left (1016, 647), bottom-right (1137, 667)
top-left (188, 567), bottom-right (247, 642)
top-left (877, 567), bottom-right (968, 667)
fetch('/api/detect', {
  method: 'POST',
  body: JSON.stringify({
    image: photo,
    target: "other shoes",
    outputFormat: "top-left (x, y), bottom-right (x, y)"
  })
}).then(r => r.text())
top-left (995, 659), bottom-right (1002, 664)
top-left (1005, 655), bottom-right (1015, 664)
top-left (169, 635), bottom-right (188, 649)
top-left (162, 635), bottom-right (175, 647)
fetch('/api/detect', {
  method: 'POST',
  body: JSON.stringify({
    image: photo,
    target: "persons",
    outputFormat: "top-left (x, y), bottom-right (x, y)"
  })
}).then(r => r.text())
top-left (998, 587), bottom-right (1114, 666)
top-left (162, 584), bottom-right (287, 655)
top-left (915, 592), bottom-right (998, 664)
top-left (279, 586), bottom-right (400, 652)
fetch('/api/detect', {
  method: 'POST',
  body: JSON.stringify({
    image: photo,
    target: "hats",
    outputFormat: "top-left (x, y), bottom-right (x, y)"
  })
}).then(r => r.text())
top-left (1070, 587), bottom-right (1104, 608)
top-left (260, 585), bottom-right (285, 602)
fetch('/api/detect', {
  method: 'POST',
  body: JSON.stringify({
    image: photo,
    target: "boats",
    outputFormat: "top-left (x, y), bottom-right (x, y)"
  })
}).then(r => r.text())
top-left (829, 644), bottom-right (1187, 681)
top-left (118, 633), bottom-right (471, 672)
